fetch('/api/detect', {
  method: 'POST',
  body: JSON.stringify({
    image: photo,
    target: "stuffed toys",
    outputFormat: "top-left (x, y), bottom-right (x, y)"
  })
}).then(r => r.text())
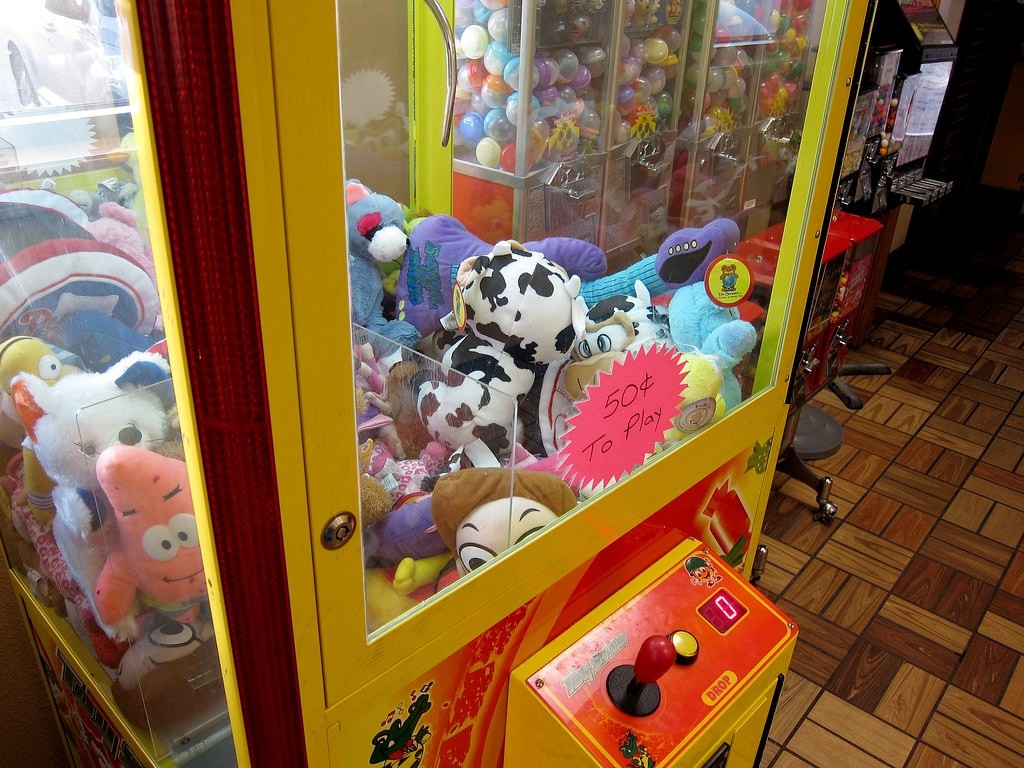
top-left (334, 173), bottom-right (761, 637)
top-left (0, 124), bottom-right (218, 736)
